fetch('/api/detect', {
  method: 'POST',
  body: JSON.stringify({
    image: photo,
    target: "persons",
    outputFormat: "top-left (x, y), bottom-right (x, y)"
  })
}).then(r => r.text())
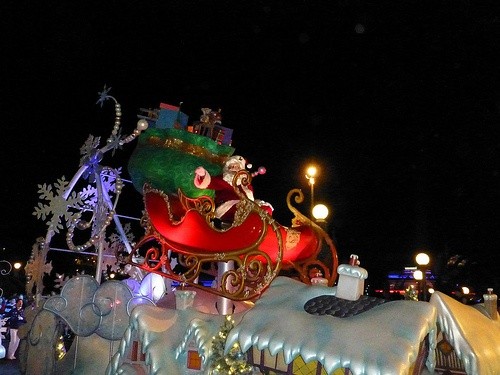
top-left (0, 297), bottom-right (6, 314)
top-left (7, 298), bottom-right (24, 359)
top-left (194, 156), bottom-right (274, 223)
top-left (3, 298), bottom-right (17, 317)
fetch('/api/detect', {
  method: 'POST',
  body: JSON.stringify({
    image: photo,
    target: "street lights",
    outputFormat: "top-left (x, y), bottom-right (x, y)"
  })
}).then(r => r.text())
top-left (13, 262), bottom-right (23, 301)
top-left (306, 165), bottom-right (320, 224)
top-left (416, 253), bottom-right (430, 303)
top-left (413, 270), bottom-right (423, 303)
